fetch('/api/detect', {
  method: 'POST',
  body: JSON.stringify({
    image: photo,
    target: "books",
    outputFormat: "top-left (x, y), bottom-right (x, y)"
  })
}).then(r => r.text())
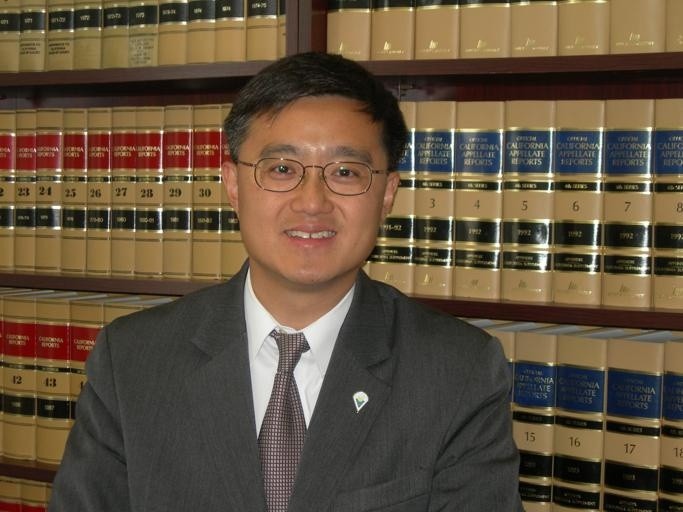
top-left (457, 317), bottom-right (682, 512)
top-left (1, 1), bottom-right (286, 72)
top-left (1, 287), bottom-right (183, 512)
top-left (328, 0), bottom-right (683, 311)
top-left (0, 104), bottom-right (250, 280)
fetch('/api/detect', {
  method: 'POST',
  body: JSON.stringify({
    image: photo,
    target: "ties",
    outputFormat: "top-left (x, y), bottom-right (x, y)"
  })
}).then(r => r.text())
top-left (258, 329), bottom-right (310, 512)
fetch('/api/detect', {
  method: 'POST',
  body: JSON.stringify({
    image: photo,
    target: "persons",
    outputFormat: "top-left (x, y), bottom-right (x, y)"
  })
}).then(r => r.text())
top-left (48, 52), bottom-right (524, 512)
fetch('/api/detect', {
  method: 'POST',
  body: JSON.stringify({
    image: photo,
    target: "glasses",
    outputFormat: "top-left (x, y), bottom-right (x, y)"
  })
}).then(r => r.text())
top-left (236, 158), bottom-right (390, 196)
